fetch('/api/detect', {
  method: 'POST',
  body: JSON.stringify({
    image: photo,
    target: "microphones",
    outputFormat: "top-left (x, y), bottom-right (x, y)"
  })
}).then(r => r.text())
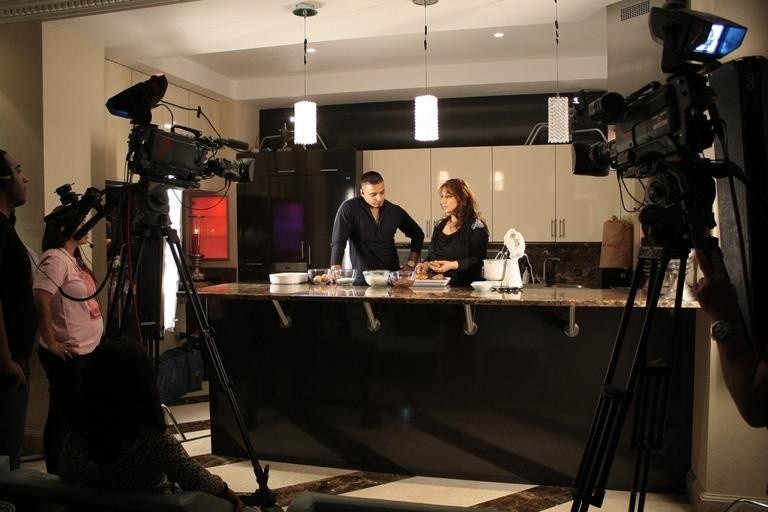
top-left (87, 241), bottom-right (93, 248)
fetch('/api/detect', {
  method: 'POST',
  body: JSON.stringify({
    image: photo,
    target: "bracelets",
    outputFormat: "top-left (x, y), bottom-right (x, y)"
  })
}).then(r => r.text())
top-left (223, 483), bottom-right (229, 498)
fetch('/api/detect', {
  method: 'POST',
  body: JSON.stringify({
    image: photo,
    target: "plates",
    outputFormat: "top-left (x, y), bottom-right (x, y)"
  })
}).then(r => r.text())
top-left (414, 277), bottom-right (451, 286)
top-left (409, 286), bottom-right (451, 294)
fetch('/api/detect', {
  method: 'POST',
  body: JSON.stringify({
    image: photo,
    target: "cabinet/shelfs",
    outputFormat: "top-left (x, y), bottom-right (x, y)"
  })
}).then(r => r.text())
top-left (492, 143), bottom-right (621, 243)
top-left (362, 145), bottom-right (493, 249)
top-left (236, 147), bottom-right (351, 281)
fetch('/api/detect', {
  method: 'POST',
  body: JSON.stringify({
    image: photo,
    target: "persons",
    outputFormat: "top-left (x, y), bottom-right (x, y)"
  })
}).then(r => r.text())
top-left (704, 29), bottom-right (714, 52)
top-left (422, 177), bottom-right (490, 286)
top-left (330, 170), bottom-right (425, 286)
top-left (59, 337), bottom-right (260, 512)
top-left (691, 248), bottom-right (768, 427)
top-left (0, 149), bottom-right (39, 470)
top-left (31, 204), bottom-right (105, 476)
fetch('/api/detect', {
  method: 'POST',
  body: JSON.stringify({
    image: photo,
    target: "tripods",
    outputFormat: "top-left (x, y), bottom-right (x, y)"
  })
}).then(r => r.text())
top-left (73, 180), bottom-right (269, 488)
top-left (570, 158), bottom-right (768, 512)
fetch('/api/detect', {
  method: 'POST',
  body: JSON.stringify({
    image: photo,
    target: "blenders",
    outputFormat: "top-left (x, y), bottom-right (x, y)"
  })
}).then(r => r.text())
top-left (470, 291), bottom-right (522, 301)
top-left (470, 227), bottom-right (527, 296)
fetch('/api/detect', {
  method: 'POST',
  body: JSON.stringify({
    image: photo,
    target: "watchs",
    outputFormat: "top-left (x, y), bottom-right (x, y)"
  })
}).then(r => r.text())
top-left (709, 321), bottom-right (742, 343)
top-left (406, 260), bottom-right (415, 268)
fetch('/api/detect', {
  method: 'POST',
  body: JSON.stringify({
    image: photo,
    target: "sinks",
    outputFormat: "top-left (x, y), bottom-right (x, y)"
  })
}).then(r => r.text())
top-left (545, 284), bottom-right (583, 289)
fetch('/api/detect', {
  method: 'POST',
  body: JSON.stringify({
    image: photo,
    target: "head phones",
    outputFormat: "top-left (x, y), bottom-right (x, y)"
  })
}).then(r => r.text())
top-left (55, 207), bottom-right (67, 235)
top-left (1, 169), bottom-right (11, 189)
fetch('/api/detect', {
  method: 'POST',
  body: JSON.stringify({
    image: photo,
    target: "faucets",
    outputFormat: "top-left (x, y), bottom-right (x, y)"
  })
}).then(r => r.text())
top-left (543, 257), bottom-right (560, 280)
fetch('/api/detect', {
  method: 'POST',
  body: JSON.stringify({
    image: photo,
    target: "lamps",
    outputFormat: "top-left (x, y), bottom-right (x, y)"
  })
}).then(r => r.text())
top-left (412, 0), bottom-right (439, 143)
top-left (291, 2), bottom-right (318, 145)
top-left (547, 0), bottom-right (573, 143)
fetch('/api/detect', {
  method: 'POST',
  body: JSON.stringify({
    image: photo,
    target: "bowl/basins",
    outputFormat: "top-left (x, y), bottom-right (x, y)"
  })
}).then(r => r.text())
top-left (269, 272), bottom-right (308, 284)
top-left (309, 286), bottom-right (413, 298)
top-left (269, 285), bottom-right (309, 294)
top-left (307, 268), bottom-right (417, 286)
